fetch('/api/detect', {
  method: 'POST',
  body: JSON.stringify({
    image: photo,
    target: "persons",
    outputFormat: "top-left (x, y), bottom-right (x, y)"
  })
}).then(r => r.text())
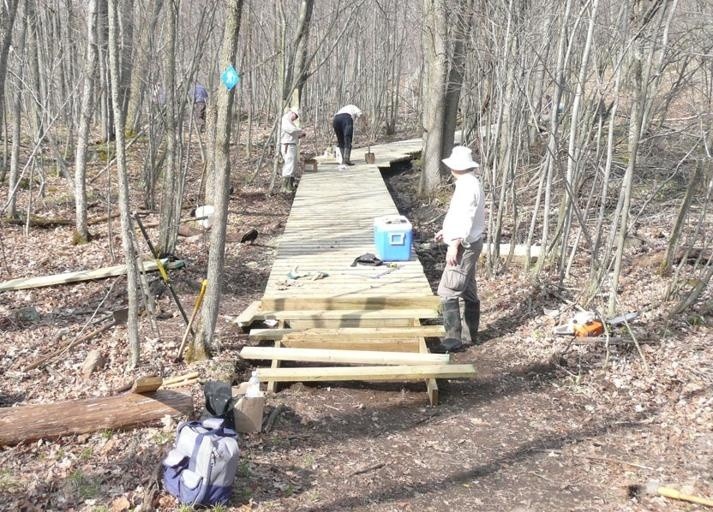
top-left (278, 106), bottom-right (305, 193)
top-left (190, 79), bottom-right (207, 133)
top-left (331, 104), bottom-right (369, 166)
top-left (425, 146), bottom-right (485, 354)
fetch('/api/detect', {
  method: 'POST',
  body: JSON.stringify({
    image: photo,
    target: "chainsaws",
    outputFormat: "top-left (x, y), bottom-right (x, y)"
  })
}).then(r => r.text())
top-left (574, 311), bottom-right (637, 337)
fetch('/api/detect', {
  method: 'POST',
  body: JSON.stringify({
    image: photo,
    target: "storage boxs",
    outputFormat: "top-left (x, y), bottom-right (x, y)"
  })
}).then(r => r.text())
top-left (304, 160), bottom-right (317, 172)
top-left (374, 216), bottom-right (412, 262)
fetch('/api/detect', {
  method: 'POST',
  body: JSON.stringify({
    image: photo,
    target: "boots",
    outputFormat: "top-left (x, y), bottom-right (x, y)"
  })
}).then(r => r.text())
top-left (430, 300), bottom-right (480, 353)
top-left (281, 176), bottom-right (297, 193)
top-left (340, 147), bottom-right (355, 165)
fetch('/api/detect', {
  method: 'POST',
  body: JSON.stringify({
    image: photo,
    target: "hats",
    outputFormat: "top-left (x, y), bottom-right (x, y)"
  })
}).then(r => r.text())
top-left (441, 146), bottom-right (479, 171)
top-left (290, 106), bottom-right (303, 119)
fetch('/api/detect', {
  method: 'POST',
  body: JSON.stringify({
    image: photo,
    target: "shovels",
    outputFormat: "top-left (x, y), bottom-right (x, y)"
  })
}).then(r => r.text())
top-left (364, 117), bottom-right (375, 164)
top-left (173, 279), bottom-right (208, 363)
top-left (22, 309), bottom-right (127, 372)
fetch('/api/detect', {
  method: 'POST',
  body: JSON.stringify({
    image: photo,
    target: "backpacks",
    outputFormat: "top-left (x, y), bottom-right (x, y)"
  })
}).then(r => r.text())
top-left (162, 418), bottom-right (240, 507)
